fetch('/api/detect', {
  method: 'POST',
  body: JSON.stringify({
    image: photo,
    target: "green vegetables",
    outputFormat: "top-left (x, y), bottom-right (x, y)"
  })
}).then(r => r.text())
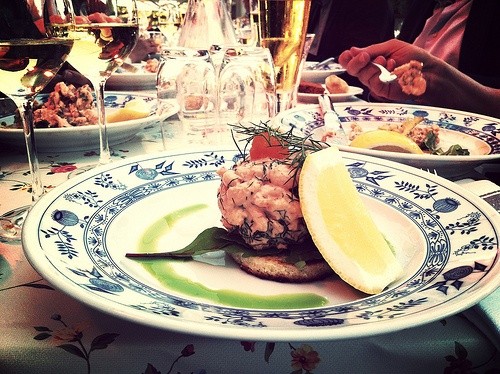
top-left (424, 130), bottom-right (471, 156)
top-left (126, 120), bottom-right (332, 270)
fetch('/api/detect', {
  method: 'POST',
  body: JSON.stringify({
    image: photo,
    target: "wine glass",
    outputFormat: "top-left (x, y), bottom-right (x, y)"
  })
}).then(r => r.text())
top-left (0, 0), bottom-right (75, 244)
top-left (41, 0), bottom-right (139, 179)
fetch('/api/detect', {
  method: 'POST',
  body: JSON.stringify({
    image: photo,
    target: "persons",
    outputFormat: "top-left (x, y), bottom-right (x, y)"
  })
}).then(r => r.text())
top-left (0, 0), bottom-right (158, 75)
top-left (306, 0), bottom-right (500, 187)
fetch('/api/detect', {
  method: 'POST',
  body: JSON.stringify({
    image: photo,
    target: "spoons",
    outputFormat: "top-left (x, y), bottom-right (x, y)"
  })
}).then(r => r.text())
top-left (370, 61), bottom-right (397, 82)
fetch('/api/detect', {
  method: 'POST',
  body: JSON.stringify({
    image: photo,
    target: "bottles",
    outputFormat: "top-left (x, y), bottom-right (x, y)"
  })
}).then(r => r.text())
top-left (176, 0), bottom-right (237, 47)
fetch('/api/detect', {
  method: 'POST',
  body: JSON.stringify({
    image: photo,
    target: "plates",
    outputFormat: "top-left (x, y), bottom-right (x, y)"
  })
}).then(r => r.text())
top-left (21, 149), bottom-right (500, 342)
top-left (297, 83), bottom-right (363, 104)
top-left (273, 102), bottom-right (500, 165)
top-left (0, 90), bottom-right (180, 153)
top-left (68, 61), bottom-right (156, 87)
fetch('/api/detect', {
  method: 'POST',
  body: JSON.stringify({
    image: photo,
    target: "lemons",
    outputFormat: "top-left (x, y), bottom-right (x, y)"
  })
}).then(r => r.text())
top-left (348, 130), bottom-right (423, 154)
top-left (298, 146), bottom-right (401, 294)
top-left (107, 98), bottom-right (150, 123)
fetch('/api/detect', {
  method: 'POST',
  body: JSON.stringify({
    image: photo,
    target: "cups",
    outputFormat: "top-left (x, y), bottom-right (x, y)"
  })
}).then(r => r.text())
top-left (265, 33), bottom-right (316, 108)
top-left (219, 47), bottom-right (276, 147)
top-left (258, 0), bottom-right (311, 115)
top-left (155, 47), bottom-right (219, 147)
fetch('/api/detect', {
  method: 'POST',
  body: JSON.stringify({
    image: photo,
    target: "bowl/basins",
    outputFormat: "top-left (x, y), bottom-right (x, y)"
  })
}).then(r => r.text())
top-left (299, 62), bottom-right (347, 83)
